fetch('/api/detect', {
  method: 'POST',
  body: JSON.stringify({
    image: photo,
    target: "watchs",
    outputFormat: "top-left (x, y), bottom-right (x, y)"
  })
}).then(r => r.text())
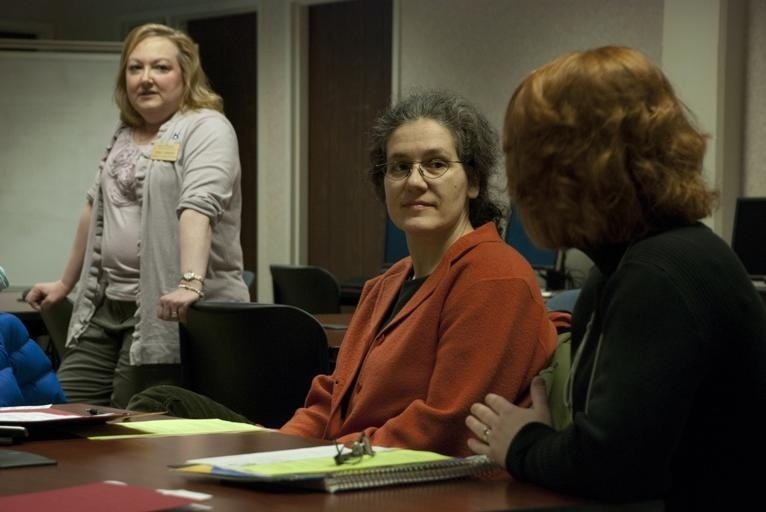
top-left (176, 271), bottom-right (205, 286)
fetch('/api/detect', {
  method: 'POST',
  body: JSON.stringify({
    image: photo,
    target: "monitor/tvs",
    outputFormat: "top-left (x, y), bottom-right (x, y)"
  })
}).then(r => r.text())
top-left (504, 208), bottom-right (566, 273)
top-left (384, 209), bottom-right (411, 266)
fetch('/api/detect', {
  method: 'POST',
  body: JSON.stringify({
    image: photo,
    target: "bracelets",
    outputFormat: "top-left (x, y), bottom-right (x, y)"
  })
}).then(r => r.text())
top-left (176, 282), bottom-right (207, 298)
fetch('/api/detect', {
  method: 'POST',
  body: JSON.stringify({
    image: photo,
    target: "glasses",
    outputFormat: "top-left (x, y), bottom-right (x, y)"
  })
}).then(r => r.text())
top-left (333, 432), bottom-right (375, 467)
top-left (373, 153), bottom-right (470, 182)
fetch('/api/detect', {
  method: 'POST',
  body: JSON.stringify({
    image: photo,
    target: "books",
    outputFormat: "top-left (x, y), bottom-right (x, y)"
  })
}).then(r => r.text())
top-left (169, 437), bottom-right (494, 493)
top-left (0, 396), bottom-right (166, 436)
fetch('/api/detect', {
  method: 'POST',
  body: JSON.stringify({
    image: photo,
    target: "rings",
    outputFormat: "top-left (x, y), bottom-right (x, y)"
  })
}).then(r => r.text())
top-left (484, 426), bottom-right (493, 434)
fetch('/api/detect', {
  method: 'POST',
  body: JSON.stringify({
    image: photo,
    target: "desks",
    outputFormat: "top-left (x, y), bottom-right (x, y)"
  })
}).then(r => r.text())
top-left (2, 402), bottom-right (664, 510)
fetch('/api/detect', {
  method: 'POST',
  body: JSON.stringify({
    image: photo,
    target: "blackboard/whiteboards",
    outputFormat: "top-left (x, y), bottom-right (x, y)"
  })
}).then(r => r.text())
top-left (0, 40), bottom-right (125, 293)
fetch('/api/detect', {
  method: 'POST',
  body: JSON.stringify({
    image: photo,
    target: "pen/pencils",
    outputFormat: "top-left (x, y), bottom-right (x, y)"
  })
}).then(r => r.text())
top-left (84, 408), bottom-right (130, 417)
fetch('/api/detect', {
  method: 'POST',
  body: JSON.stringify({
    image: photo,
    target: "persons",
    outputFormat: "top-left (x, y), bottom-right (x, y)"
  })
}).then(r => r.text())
top-left (460, 45), bottom-right (762, 509)
top-left (23, 24), bottom-right (254, 413)
top-left (124, 89), bottom-right (560, 458)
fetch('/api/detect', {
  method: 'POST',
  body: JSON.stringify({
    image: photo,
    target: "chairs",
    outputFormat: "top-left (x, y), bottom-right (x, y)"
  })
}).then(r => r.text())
top-left (270, 266), bottom-right (342, 313)
top-left (382, 193), bottom-right (567, 291)
top-left (177, 303), bottom-right (332, 427)
top-left (33, 297), bottom-right (74, 362)
